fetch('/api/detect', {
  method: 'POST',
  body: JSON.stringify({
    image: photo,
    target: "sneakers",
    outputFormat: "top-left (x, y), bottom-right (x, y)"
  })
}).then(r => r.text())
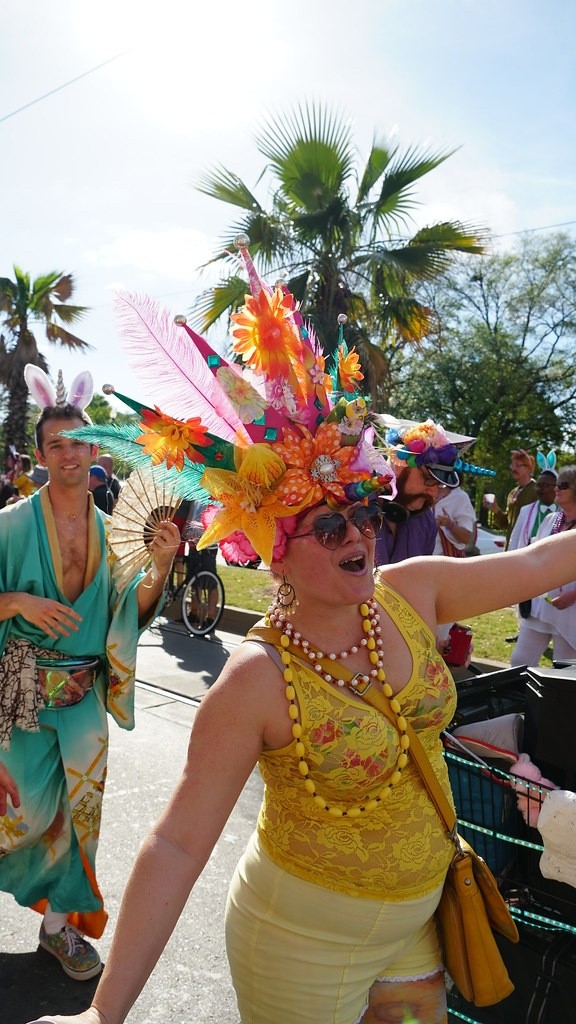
top-left (39, 918), bottom-right (101, 980)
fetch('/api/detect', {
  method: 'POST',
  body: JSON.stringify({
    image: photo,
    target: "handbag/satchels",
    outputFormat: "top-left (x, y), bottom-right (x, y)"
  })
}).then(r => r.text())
top-left (438, 834), bottom-right (520, 1008)
top-left (441, 536), bottom-right (466, 558)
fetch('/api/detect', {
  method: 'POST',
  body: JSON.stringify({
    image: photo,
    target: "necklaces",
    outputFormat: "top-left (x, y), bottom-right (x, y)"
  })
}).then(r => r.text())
top-left (549, 512), bottom-right (562, 535)
top-left (268, 599), bottom-right (409, 817)
top-left (525, 500), bottom-right (560, 540)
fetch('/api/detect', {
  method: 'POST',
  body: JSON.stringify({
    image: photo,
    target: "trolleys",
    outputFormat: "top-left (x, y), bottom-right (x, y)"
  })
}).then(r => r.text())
top-left (419, 654), bottom-right (576, 1024)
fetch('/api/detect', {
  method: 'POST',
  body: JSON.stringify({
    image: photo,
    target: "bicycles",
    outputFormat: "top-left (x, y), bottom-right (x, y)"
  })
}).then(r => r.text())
top-left (157, 548), bottom-right (225, 636)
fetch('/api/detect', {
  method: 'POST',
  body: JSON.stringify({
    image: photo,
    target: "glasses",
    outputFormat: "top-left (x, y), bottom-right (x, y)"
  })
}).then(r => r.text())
top-left (286, 502), bottom-right (385, 551)
top-left (418, 468), bottom-right (447, 489)
top-left (535, 483), bottom-right (555, 489)
top-left (510, 464), bottom-right (527, 469)
top-left (555, 482), bottom-right (569, 490)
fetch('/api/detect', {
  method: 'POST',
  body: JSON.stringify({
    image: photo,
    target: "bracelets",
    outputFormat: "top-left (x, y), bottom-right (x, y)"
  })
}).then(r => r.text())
top-left (447, 522), bottom-right (454, 529)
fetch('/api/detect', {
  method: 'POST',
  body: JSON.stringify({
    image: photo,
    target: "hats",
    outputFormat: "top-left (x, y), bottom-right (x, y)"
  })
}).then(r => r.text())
top-left (25, 464), bottom-right (49, 484)
top-left (377, 421), bottom-right (496, 489)
top-left (89, 465), bottom-right (107, 478)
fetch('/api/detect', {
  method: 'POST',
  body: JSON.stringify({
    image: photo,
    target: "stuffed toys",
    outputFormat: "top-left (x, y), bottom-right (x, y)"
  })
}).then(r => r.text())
top-left (509, 754), bottom-right (558, 828)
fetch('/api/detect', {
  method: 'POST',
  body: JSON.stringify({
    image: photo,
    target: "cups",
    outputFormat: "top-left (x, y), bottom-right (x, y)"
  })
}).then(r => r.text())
top-left (484, 494), bottom-right (495, 507)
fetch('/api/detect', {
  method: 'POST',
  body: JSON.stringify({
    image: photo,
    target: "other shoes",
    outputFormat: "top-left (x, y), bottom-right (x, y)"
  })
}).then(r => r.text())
top-left (505, 634), bottom-right (518, 642)
top-left (196, 618), bottom-right (215, 637)
top-left (174, 613), bottom-right (199, 624)
top-left (175, 586), bottom-right (186, 599)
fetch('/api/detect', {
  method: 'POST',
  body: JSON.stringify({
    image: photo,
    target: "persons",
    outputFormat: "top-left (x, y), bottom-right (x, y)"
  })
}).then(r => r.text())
top-left (482, 452), bottom-right (538, 552)
top-left (88, 454), bottom-right (218, 631)
top-left (369, 421), bottom-right (477, 652)
top-left (30, 491), bottom-right (576, 1024)
top-left (0, 405), bottom-right (181, 979)
top-left (511, 466), bottom-right (576, 668)
top-left (505, 470), bottom-right (563, 642)
top-left (0, 445), bottom-right (49, 508)
